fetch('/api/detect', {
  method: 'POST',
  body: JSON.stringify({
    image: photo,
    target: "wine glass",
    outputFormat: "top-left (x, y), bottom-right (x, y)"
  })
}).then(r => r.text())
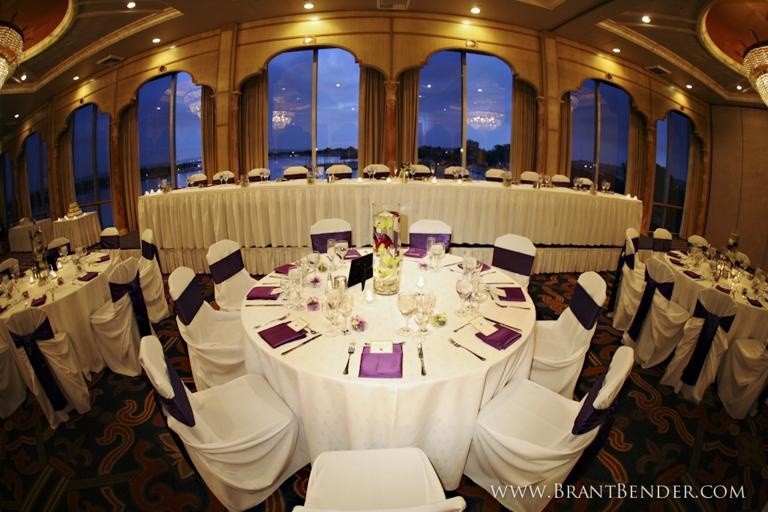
top-left (0, 246), bottom-right (105, 314)
top-left (153, 162), bottom-right (611, 195)
top-left (688, 232), bottom-right (768, 307)
top-left (282, 237), bottom-right (495, 338)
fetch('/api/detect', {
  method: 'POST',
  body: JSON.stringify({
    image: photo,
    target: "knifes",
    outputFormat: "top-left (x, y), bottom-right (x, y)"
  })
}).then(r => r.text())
top-left (281, 334), bottom-right (322, 354)
top-left (480, 281), bottom-right (515, 286)
top-left (417, 343), bottom-right (428, 376)
top-left (483, 316), bottom-right (522, 332)
top-left (244, 302), bottom-right (285, 308)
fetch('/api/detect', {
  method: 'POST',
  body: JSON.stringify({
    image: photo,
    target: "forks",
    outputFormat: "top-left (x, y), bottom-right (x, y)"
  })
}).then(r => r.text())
top-left (495, 302), bottom-right (531, 310)
top-left (446, 337), bottom-right (486, 362)
top-left (342, 341), bottom-right (356, 374)
top-left (253, 312), bottom-right (290, 330)
